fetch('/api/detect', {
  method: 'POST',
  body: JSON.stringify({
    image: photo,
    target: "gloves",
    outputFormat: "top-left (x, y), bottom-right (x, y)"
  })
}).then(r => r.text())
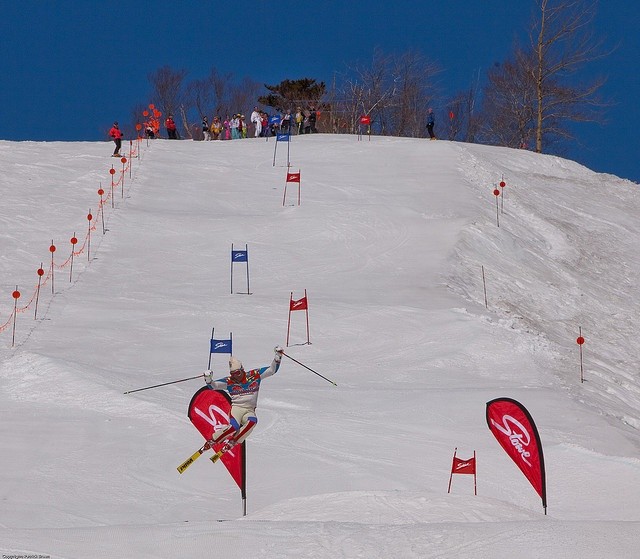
top-left (274, 345), bottom-right (284, 362)
top-left (204, 370), bottom-right (213, 384)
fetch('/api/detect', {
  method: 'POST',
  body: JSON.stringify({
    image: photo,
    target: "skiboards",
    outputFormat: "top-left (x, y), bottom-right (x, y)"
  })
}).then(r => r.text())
top-left (177, 440), bottom-right (226, 474)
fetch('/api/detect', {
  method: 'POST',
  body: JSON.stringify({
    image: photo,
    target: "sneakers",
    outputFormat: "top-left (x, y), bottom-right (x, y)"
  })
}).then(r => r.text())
top-left (203, 439), bottom-right (216, 450)
top-left (222, 439), bottom-right (236, 454)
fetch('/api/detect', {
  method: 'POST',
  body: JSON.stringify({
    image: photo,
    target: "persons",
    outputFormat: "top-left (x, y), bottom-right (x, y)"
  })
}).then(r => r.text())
top-left (164, 114), bottom-right (181, 141)
top-left (426, 106), bottom-right (438, 142)
top-left (199, 104), bottom-right (317, 141)
top-left (109, 120), bottom-right (126, 159)
top-left (204, 347), bottom-right (284, 452)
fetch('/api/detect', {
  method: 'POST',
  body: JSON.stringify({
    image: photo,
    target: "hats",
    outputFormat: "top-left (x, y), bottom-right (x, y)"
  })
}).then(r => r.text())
top-left (228, 357), bottom-right (243, 372)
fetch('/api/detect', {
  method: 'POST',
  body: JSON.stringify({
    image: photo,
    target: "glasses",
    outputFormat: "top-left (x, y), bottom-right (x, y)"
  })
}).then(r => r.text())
top-left (229, 370), bottom-right (242, 375)
